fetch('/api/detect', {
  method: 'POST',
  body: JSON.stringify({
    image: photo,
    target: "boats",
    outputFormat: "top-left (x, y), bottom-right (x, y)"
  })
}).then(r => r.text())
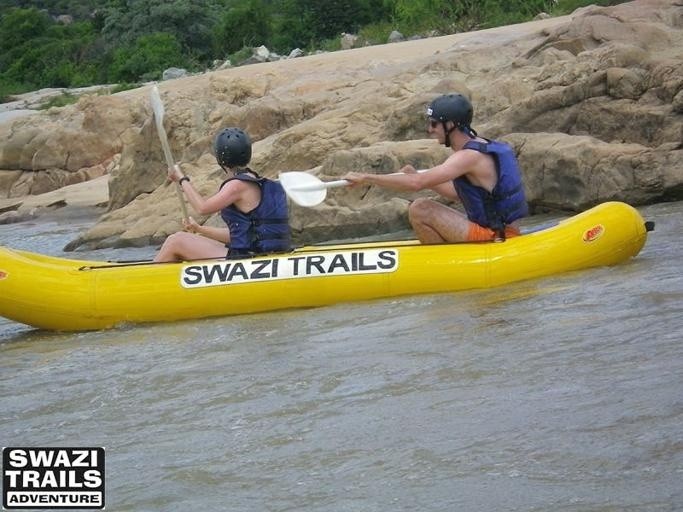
top-left (0, 199), bottom-right (665, 334)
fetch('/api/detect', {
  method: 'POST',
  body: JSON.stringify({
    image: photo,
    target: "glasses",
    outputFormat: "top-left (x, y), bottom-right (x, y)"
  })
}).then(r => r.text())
top-left (430, 119), bottom-right (443, 128)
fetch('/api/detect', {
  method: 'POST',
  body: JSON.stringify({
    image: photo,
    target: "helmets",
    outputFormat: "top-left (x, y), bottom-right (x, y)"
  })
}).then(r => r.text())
top-left (428, 95), bottom-right (475, 126)
top-left (214, 129), bottom-right (252, 164)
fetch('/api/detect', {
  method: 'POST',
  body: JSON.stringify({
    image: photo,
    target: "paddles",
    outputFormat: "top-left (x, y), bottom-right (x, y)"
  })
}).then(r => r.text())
top-left (279, 168), bottom-right (432, 207)
top-left (149, 85), bottom-right (194, 233)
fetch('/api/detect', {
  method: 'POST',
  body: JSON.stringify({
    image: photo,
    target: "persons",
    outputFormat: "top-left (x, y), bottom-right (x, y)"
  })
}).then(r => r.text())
top-left (341, 96), bottom-right (528, 244)
top-left (155, 126), bottom-right (292, 260)
